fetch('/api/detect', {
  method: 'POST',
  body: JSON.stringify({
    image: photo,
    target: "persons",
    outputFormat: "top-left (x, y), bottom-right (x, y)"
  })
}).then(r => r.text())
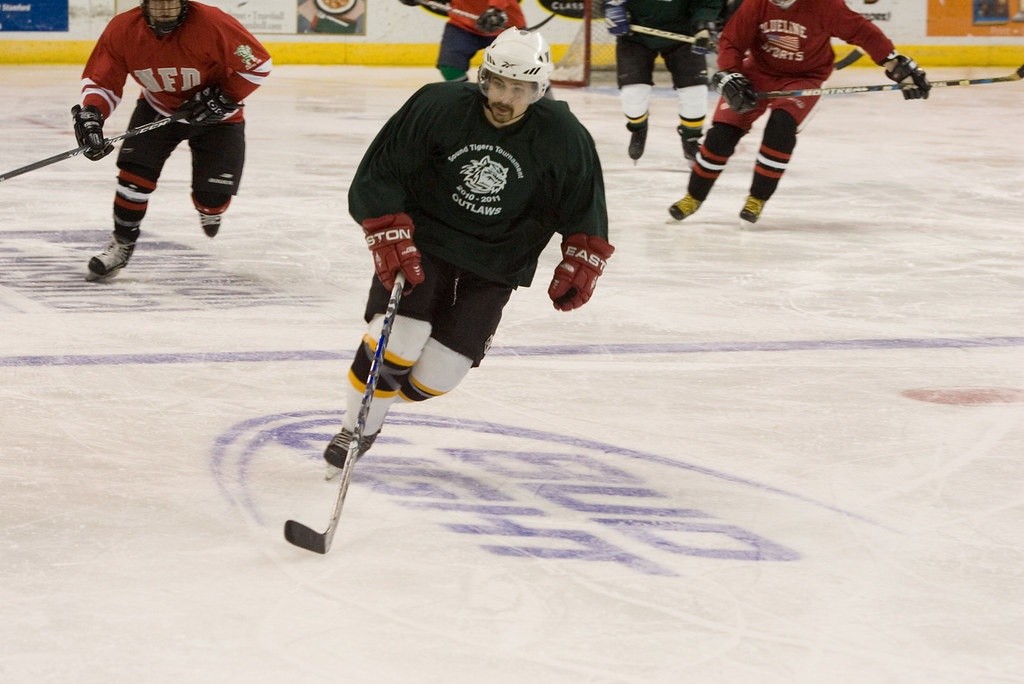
top-left (323, 26), bottom-right (617, 480)
top-left (665, 0), bottom-right (930, 229)
top-left (602, 0), bottom-right (740, 171)
top-left (70, 1), bottom-right (272, 283)
top-left (399, 0), bottom-right (528, 81)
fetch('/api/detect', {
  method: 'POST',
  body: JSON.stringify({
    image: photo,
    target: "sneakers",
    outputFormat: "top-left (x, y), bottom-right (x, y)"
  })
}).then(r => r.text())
top-left (200, 212), bottom-right (220, 237)
top-left (324, 428), bottom-right (381, 479)
top-left (627, 120), bottom-right (647, 165)
top-left (740, 195), bottom-right (767, 223)
top-left (86, 229), bottom-right (140, 281)
top-left (669, 194), bottom-right (702, 221)
top-left (677, 125), bottom-right (705, 161)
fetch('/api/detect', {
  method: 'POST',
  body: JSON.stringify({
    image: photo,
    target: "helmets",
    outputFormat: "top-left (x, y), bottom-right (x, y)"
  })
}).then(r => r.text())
top-left (141, 0), bottom-right (187, 36)
top-left (478, 25), bottom-right (551, 104)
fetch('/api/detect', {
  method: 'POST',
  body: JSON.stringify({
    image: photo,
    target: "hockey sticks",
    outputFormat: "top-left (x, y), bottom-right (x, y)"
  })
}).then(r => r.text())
top-left (416, 0), bottom-right (555, 36)
top-left (0, 111), bottom-right (184, 184)
top-left (627, 23), bottom-right (863, 74)
top-left (752, 63), bottom-right (1024, 99)
top-left (281, 269), bottom-right (408, 555)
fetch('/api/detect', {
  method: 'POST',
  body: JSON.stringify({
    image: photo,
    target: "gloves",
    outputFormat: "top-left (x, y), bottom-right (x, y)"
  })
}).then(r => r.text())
top-left (603, 0), bottom-right (632, 36)
top-left (549, 232), bottom-right (614, 311)
top-left (691, 20), bottom-right (717, 54)
top-left (178, 83), bottom-right (235, 128)
top-left (71, 103), bottom-right (114, 161)
top-left (476, 8), bottom-right (504, 33)
top-left (885, 52), bottom-right (931, 101)
top-left (362, 213), bottom-right (424, 296)
top-left (713, 71), bottom-right (757, 114)
